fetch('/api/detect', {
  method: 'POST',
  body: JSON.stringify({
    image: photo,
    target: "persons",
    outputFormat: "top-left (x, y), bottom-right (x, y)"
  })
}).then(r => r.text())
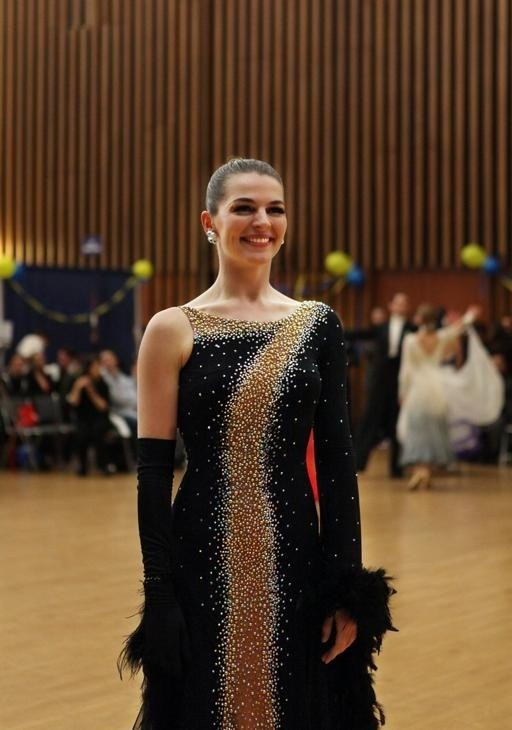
top-left (115, 156), bottom-right (400, 730)
top-left (0, 331), bottom-right (186, 477)
top-left (341, 290), bottom-right (512, 492)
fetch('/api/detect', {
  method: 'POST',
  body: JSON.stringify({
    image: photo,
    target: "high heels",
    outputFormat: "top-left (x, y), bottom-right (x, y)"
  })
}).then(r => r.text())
top-left (406, 467), bottom-right (432, 492)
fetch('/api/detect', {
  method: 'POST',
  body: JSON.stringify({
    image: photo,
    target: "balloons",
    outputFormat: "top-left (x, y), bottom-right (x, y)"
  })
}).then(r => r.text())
top-left (484, 256), bottom-right (498, 272)
top-left (345, 264), bottom-right (364, 287)
top-left (462, 243), bottom-right (485, 269)
top-left (130, 258), bottom-right (154, 281)
top-left (0, 253), bottom-right (15, 280)
top-left (324, 250), bottom-right (352, 277)
